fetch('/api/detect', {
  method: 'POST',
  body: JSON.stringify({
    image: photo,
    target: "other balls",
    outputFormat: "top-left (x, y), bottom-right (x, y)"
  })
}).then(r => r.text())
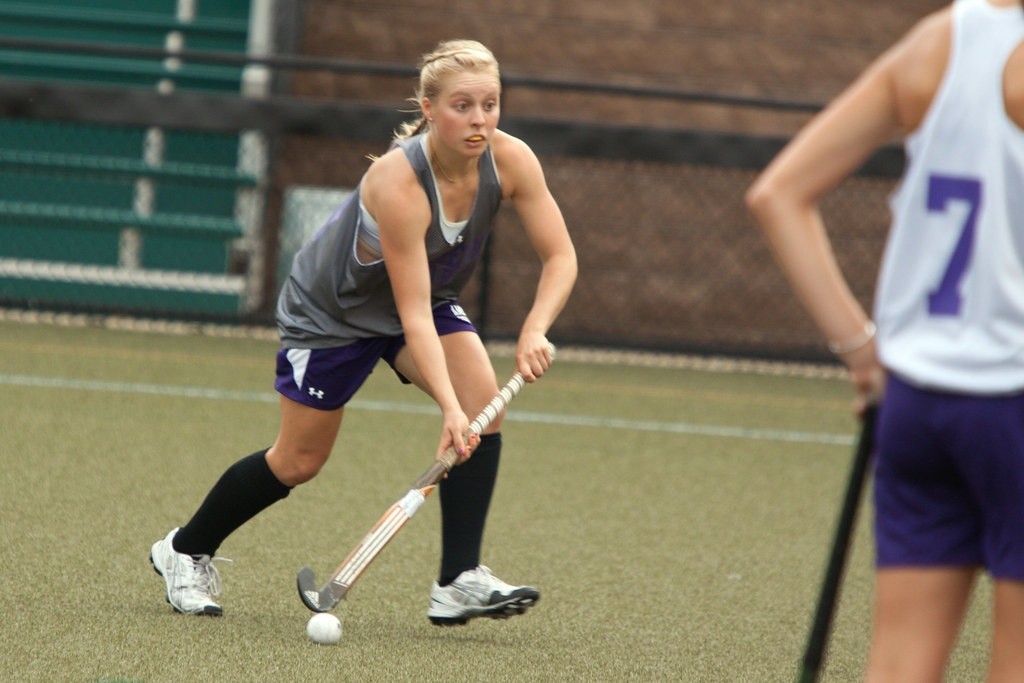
top-left (307, 613), bottom-right (343, 645)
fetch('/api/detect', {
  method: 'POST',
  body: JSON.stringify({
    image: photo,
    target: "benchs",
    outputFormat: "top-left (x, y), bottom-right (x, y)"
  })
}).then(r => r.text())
top-left (0, 0), bottom-right (275, 316)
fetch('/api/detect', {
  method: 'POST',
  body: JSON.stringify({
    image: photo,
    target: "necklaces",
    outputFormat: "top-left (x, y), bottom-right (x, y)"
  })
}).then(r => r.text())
top-left (433, 153), bottom-right (475, 182)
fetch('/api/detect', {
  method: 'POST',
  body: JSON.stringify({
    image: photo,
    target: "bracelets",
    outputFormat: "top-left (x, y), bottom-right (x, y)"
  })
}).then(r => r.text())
top-left (826, 323), bottom-right (878, 357)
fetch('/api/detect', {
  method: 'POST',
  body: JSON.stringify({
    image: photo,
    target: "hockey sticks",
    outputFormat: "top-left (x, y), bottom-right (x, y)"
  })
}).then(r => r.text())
top-left (295, 343), bottom-right (556, 613)
top-left (794, 395), bottom-right (878, 683)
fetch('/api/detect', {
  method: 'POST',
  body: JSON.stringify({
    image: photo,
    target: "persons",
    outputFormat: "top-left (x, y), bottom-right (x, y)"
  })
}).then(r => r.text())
top-left (745, 2), bottom-right (1024, 682)
top-left (149, 39), bottom-right (581, 626)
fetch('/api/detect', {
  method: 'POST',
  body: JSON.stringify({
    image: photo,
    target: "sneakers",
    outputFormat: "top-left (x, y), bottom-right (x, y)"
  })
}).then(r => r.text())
top-left (426, 564), bottom-right (540, 627)
top-left (149, 526), bottom-right (233, 616)
